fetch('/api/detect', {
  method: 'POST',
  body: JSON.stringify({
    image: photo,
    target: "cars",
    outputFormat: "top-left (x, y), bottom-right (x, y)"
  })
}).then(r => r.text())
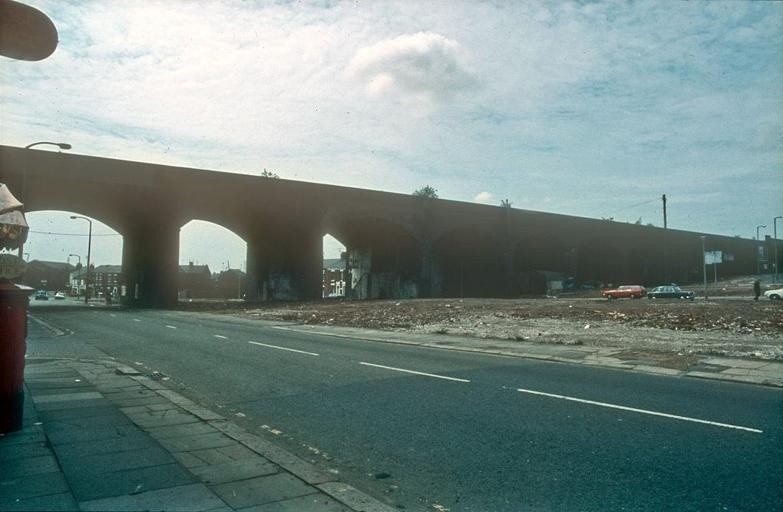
top-left (648, 285), bottom-right (694, 300)
top-left (54, 293), bottom-right (64, 299)
top-left (35, 290), bottom-right (47, 299)
top-left (764, 287), bottom-right (783, 299)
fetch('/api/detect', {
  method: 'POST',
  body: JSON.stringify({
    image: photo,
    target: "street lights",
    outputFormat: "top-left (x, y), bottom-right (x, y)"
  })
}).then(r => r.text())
top-left (756, 223), bottom-right (767, 276)
top-left (68, 216), bottom-right (93, 304)
top-left (774, 216), bottom-right (783, 280)
top-left (68, 254), bottom-right (80, 301)
top-left (18, 141), bottom-right (71, 261)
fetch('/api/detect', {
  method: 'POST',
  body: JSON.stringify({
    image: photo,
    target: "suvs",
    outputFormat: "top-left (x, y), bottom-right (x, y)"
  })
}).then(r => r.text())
top-left (602, 283), bottom-right (648, 300)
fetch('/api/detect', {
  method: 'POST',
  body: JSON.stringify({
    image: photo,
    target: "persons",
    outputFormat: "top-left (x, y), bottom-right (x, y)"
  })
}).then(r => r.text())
top-left (753, 278), bottom-right (761, 301)
